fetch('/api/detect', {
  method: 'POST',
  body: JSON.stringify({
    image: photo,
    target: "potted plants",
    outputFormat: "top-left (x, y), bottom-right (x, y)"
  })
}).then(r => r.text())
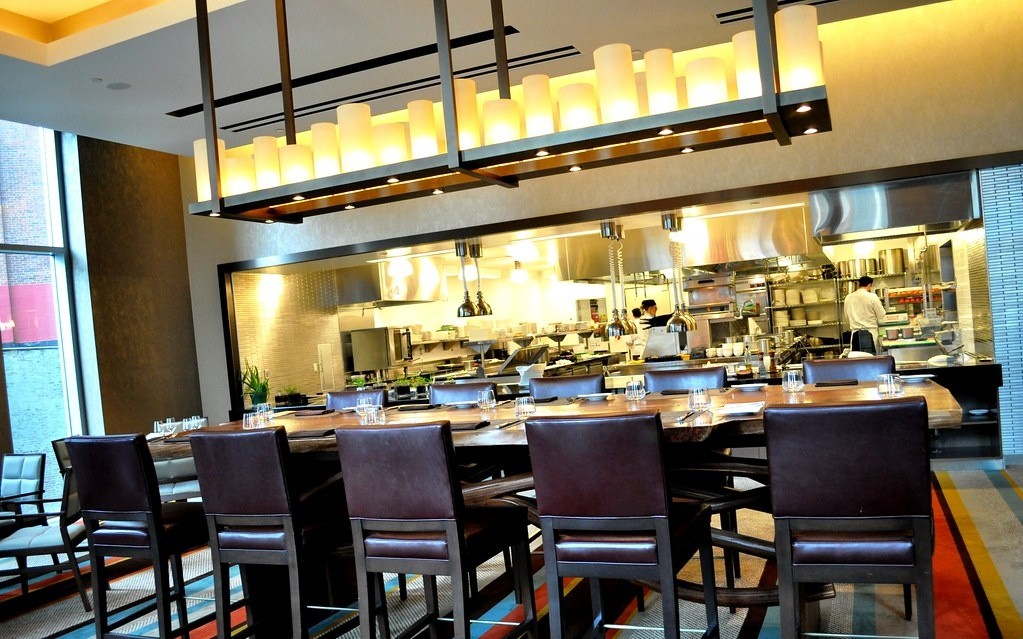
top-left (392, 377), bottom-right (411, 395)
top-left (414, 376), bottom-right (429, 395)
top-left (283, 384), bottom-right (302, 405)
top-left (237, 357), bottom-right (273, 406)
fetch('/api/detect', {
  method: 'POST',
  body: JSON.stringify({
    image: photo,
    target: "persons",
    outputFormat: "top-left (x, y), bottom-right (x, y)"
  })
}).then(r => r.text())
top-left (844, 276), bottom-right (888, 357)
top-left (631, 299), bottom-right (657, 334)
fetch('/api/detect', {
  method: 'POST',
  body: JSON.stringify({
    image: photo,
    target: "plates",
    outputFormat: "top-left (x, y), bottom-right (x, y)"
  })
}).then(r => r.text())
top-left (716, 383), bottom-right (766, 415)
top-left (578, 393), bottom-right (611, 401)
top-left (773, 289), bottom-right (785, 306)
top-left (773, 309), bottom-right (825, 327)
top-left (445, 402), bottom-right (478, 408)
top-left (896, 373), bottom-right (934, 384)
top-left (786, 289), bottom-right (799, 304)
top-left (801, 287), bottom-right (821, 303)
top-left (969, 410), bottom-right (989, 416)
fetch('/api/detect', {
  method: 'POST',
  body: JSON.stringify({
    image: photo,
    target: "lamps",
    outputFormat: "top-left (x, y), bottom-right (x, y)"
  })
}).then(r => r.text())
top-left (452, 241), bottom-right (495, 318)
top-left (165, 0), bottom-right (832, 225)
top-left (597, 221), bottom-right (637, 336)
top-left (660, 213), bottom-right (698, 334)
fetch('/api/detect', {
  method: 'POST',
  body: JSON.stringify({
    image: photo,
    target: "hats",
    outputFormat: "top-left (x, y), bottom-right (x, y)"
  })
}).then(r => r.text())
top-left (642, 299), bottom-right (657, 309)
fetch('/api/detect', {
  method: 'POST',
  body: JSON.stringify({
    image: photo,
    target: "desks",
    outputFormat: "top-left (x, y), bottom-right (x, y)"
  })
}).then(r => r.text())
top-left (150, 375), bottom-right (962, 608)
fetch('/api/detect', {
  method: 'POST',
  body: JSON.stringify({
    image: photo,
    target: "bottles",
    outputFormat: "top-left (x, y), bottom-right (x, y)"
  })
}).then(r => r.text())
top-left (744, 346), bottom-right (776, 376)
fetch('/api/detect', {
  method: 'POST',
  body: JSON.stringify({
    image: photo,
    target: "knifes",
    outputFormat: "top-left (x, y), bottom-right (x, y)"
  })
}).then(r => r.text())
top-left (677, 411), bottom-right (694, 422)
top-left (494, 400), bottom-right (520, 428)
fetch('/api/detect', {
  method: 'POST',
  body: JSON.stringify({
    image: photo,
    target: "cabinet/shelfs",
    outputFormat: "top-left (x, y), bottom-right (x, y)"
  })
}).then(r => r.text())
top-left (767, 268), bottom-right (914, 366)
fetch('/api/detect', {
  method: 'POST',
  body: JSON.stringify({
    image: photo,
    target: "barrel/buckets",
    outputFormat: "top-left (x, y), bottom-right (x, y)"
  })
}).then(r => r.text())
top-left (839, 249), bottom-right (903, 300)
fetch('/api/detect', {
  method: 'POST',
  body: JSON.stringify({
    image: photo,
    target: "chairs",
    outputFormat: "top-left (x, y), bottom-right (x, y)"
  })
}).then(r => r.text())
top-left (0, 353), bottom-right (942, 639)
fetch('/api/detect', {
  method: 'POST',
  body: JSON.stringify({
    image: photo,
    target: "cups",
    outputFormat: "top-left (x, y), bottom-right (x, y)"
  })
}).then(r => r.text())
top-left (477, 390), bottom-right (497, 410)
top-left (183, 415), bottom-right (201, 430)
top-left (153, 417), bottom-right (176, 434)
top-left (781, 370), bottom-right (805, 392)
top-left (243, 403), bottom-right (275, 429)
top-left (688, 387), bottom-right (712, 412)
top-left (625, 380), bottom-right (645, 400)
top-left (706, 335), bottom-right (770, 357)
top-left (515, 396), bottom-right (536, 420)
top-left (877, 374), bottom-right (902, 399)
top-left (822, 264), bottom-right (833, 280)
top-left (357, 398), bottom-right (386, 425)
